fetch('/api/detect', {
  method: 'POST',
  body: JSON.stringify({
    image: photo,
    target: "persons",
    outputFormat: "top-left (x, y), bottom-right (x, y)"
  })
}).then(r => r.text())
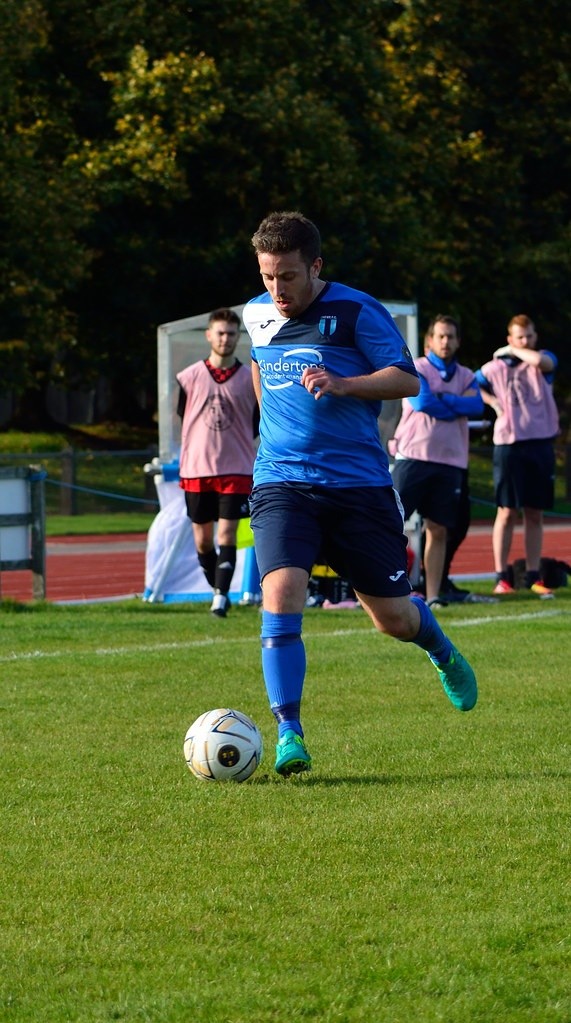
top-left (475, 314), bottom-right (561, 593)
top-left (410, 335), bottom-right (472, 595)
top-left (176, 309), bottom-right (261, 617)
top-left (390, 316), bottom-right (486, 608)
top-left (243, 212), bottom-right (478, 777)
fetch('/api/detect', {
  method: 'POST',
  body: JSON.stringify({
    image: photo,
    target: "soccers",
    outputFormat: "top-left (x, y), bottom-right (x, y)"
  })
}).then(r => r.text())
top-left (184, 708), bottom-right (263, 783)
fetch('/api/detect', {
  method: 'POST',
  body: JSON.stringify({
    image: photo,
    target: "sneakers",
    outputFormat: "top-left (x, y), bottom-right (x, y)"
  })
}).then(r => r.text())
top-left (210, 593), bottom-right (229, 617)
top-left (431, 645), bottom-right (478, 712)
top-left (276, 732), bottom-right (312, 776)
top-left (494, 577), bottom-right (516, 594)
top-left (531, 583), bottom-right (555, 599)
